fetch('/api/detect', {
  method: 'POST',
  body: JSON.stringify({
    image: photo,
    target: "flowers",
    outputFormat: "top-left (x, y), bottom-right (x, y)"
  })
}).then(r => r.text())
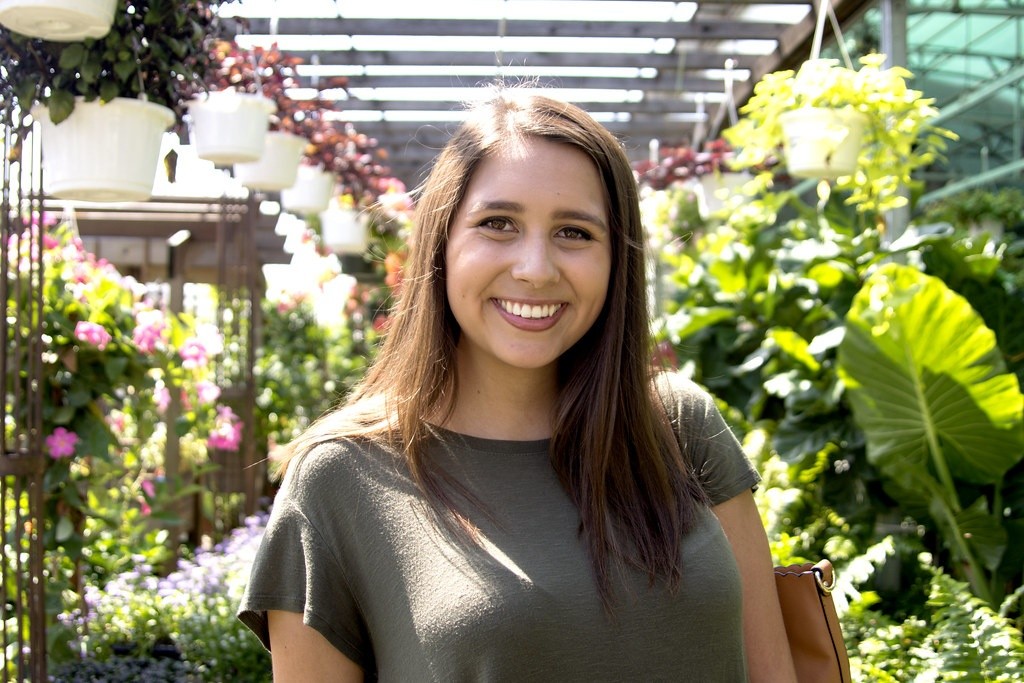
top-left (0, 209), bottom-right (247, 544)
top-left (53, 506), bottom-right (276, 683)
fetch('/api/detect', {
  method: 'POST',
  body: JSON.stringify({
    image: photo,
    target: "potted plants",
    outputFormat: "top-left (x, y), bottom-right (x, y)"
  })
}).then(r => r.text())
top-left (0, 0), bottom-right (1024, 558)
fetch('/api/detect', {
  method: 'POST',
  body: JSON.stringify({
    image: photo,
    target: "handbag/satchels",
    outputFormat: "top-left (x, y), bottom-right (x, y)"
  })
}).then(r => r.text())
top-left (773, 559), bottom-right (853, 683)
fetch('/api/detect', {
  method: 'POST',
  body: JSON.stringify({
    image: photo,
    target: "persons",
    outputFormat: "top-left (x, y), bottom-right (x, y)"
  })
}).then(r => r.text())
top-left (238, 92), bottom-right (794, 683)
